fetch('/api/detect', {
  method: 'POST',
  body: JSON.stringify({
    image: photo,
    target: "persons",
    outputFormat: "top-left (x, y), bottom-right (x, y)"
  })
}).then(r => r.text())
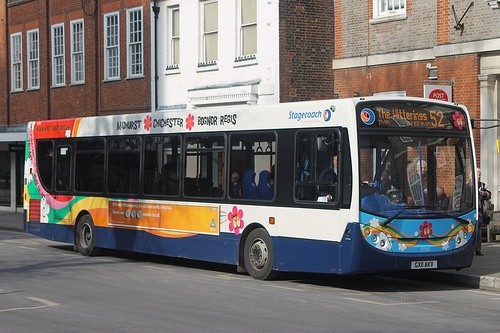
top-left (317, 152), bottom-right (338, 201)
top-left (408, 159), bottom-right (449, 211)
top-left (463, 168), bottom-right (492, 256)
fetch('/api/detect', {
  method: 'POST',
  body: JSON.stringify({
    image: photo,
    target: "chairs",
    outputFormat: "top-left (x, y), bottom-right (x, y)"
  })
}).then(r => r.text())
top-left (240, 169), bottom-right (274, 201)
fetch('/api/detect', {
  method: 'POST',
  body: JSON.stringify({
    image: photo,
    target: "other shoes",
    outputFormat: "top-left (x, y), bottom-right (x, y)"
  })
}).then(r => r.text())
top-left (476, 251), bottom-right (485, 255)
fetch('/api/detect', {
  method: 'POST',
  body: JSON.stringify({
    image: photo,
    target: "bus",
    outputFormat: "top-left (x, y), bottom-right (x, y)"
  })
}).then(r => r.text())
top-left (23, 97), bottom-right (479, 279)
